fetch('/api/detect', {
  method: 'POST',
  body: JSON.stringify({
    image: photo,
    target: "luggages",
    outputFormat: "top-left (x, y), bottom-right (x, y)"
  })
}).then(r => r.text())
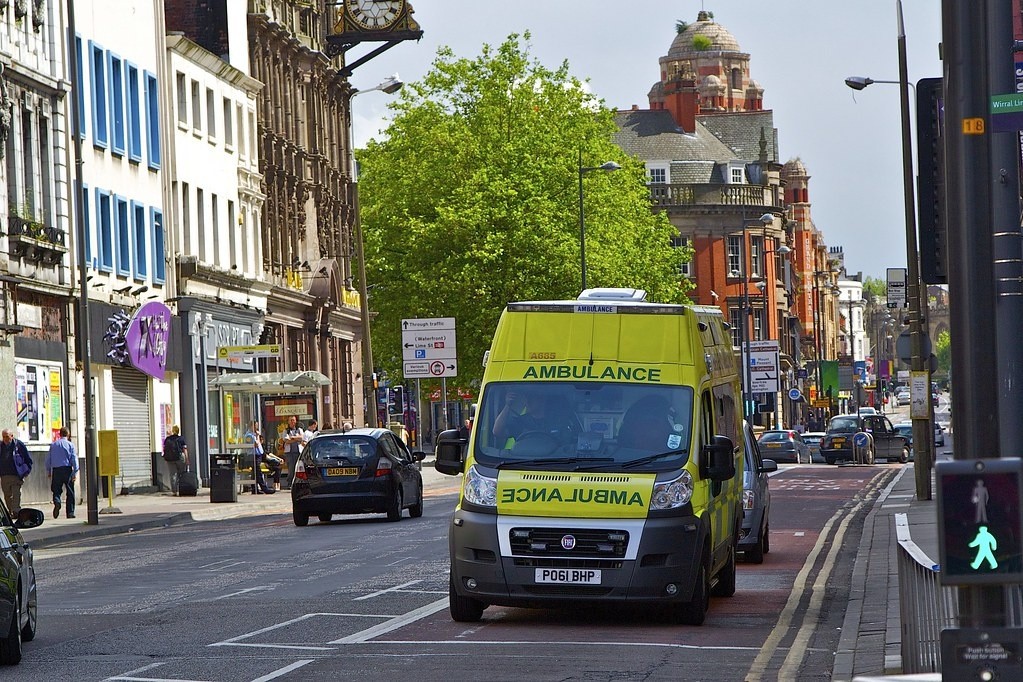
top-left (179, 463), bottom-right (197, 497)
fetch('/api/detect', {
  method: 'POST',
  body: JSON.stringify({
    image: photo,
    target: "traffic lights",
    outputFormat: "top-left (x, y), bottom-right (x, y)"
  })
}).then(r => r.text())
top-left (881, 379), bottom-right (887, 392)
top-left (937, 456), bottom-right (1022, 586)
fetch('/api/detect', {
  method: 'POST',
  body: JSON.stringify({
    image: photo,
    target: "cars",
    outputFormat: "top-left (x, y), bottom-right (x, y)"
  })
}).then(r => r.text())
top-left (756, 407), bottom-right (946, 466)
top-left (736, 419), bottom-right (779, 564)
top-left (290, 427), bottom-right (426, 527)
top-left (893, 381), bottom-right (940, 407)
top-left (0, 498), bottom-right (44, 665)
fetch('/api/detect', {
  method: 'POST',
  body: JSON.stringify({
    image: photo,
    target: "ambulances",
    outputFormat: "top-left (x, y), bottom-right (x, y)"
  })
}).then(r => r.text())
top-left (448, 286), bottom-right (745, 625)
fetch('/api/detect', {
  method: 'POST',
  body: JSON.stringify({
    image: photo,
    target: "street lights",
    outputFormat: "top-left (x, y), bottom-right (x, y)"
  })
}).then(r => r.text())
top-left (844, 76), bottom-right (916, 127)
top-left (814, 257), bottom-right (840, 431)
top-left (347, 72), bottom-right (405, 429)
top-left (848, 290), bottom-right (868, 376)
top-left (759, 213), bottom-right (791, 341)
top-left (579, 146), bottom-right (621, 290)
top-left (875, 309), bottom-right (896, 412)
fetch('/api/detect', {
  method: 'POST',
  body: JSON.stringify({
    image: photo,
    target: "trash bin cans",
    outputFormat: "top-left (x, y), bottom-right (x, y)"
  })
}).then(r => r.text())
top-left (210, 453), bottom-right (238, 503)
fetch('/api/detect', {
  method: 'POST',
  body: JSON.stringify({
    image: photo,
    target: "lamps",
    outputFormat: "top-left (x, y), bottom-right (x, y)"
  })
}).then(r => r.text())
top-left (263, 257), bottom-right (329, 279)
top-left (238, 214), bottom-right (243, 225)
top-left (216, 297), bottom-right (272, 316)
top-left (356, 373), bottom-right (361, 381)
top-left (78, 276), bottom-right (182, 302)
top-left (309, 328), bottom-right (332, 339)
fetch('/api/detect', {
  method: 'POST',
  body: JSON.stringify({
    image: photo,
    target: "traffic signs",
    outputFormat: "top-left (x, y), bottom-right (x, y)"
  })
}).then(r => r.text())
top-left (401, 317), bottom-right (456, 330)
top-left (402, 330), bottom-right (456, 361)
top-left (402, 358), bottom-right (458, 378)
top-left (742, 341), bottom-right (781, 394)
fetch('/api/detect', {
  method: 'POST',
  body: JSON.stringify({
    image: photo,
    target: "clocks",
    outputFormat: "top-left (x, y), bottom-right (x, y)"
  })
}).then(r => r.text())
top-left (325, 0), bottom-right (425, 78)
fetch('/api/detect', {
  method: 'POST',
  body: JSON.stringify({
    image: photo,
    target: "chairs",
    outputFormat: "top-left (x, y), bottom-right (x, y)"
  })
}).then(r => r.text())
top-left (616, 395), bottom-right (681, 456)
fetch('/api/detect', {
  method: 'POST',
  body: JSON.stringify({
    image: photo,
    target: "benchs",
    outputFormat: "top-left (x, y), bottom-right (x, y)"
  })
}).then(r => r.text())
top-left (235, 461), bottom-right (289, 496)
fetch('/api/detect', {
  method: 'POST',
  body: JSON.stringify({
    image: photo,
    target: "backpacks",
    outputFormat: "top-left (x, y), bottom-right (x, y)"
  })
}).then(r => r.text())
top-left (164, 436), bottom-right (182, 461)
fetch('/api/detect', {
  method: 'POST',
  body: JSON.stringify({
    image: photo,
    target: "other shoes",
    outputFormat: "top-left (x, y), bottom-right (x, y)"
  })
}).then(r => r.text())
top-left (67, 515), bottom-right (75, 518)
top-left (266, 490), bottom-right (275, 494)
top-left (252, 491), bottom-right (264, 494)
top-left (53, 502), bottom-right (61, 518)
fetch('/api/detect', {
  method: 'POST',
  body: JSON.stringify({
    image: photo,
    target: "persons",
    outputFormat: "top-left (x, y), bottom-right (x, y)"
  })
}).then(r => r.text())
top-left (848, 399), bottom-right (880, 414)
top-left (18, 510), bottom-right (37, 525)
top-left (885, 389), bottom-right (890, 400)
top-left (784, 406), bottom-right (830, 434)
top-left (425, 427), bottom-right (432, 443)
top-left (164, 425), bottom-right (190, 497)
top-left (0, 429), bottom-right (33, 519)
top-left (364, 422), bottom-right (409, 445)
top-left (493, 392), bottom-right (561, 442)
top-left (322, 421), bottom-right (332, 430)
top-left (244, 421), bottom-right (284, 495)
top-left (460, 420), bottom-right (470, 457)
top-left (45, 427), bottom-right (80, 518)
top-left (343, 423), bottom-right (352, 429)
top-left (949, 415), bottom-right (953, 436)
top-left (283, 417), bottom-right (318, 489)
